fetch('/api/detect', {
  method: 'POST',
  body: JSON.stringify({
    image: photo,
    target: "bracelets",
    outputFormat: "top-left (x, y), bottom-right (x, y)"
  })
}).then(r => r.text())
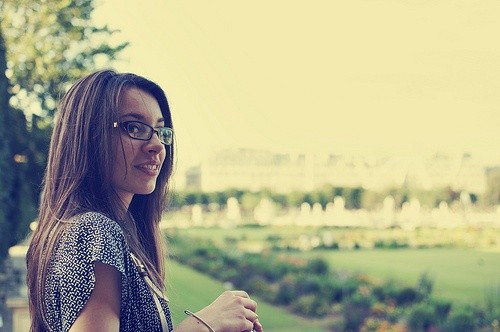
top-left (185, 309), bottom-right (215, 331)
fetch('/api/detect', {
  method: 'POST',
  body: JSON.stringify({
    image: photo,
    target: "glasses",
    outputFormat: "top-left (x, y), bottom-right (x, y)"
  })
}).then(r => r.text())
top-left (113, 120), bottom-right (175, 147)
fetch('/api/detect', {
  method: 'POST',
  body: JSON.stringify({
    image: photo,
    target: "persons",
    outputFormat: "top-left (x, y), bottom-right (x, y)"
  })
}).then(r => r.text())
top-left (25, 69), bottom-right (263, 332)
